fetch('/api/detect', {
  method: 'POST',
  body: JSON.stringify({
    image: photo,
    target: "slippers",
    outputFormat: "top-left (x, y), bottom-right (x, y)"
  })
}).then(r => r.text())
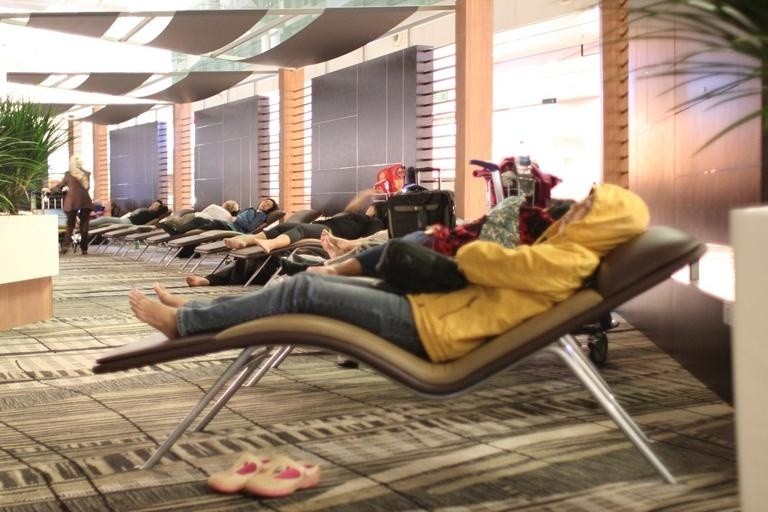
top-left (208, 453), bottom-right (292, 493)
top-left (245, 454), bottom-right (318, 497)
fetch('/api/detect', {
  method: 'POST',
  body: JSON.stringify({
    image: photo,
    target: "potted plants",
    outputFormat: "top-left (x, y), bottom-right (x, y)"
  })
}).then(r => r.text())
top-left (0, 93), bottom-right (82, 285)
top-left (613, 1), bottom-right (768, 512)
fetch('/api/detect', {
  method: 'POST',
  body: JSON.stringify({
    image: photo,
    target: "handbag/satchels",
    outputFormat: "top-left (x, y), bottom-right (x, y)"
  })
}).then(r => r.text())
top-left (388, 192), bottom-right (455, 238)
top-left (376, 238), bottom-right (467, 293)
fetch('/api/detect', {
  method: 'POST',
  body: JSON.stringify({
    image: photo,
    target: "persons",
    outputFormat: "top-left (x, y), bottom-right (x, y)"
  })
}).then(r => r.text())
top-left (88, 155), bottom-right (560, 279)
top-left (129, 181), bottom-right (650, 365)
top-left (40, 154), bottom-right (91, 255)
